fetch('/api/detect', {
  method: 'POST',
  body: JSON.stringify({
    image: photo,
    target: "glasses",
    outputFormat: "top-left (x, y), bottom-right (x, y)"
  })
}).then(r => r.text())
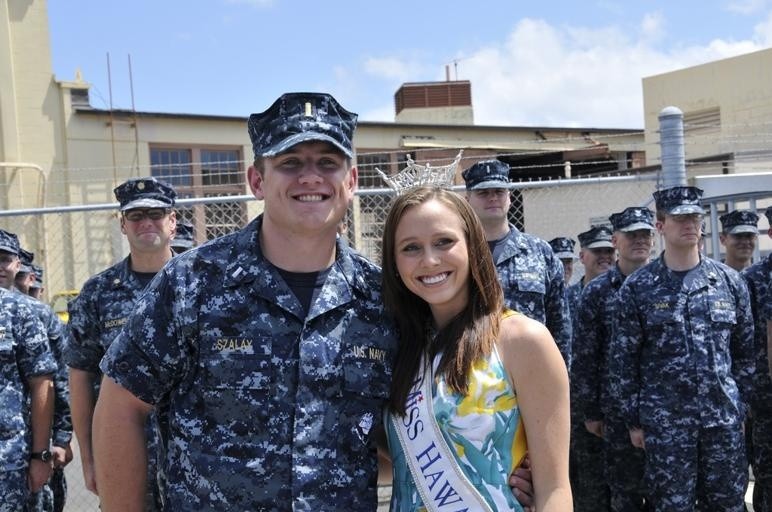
top-left (122, 208), bottom-right (178, 222)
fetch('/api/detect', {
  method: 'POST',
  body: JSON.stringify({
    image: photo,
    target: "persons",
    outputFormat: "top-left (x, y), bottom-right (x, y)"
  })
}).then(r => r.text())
top-left (373, 147), bottom-right (576, 511)
top-left (90, 93), bottom-right (534, 511)
top-left (67, 179), bottom-right (179, 494)
top-left (571, 206), bottom-right (655, 511)
top-left (739, 206), bottom-right (772, 511)
top-left (462, 157), bottom-right (576, 379)
top-left (0, 225), bottom-right (73, 512)
top-left (549, 235), bottom-right (575, 284)
top-left (715, 210), bottom-right (759, 270)
top-left (564, 225), bottom-right (616, 335)
top-left (607, 187), bottom-right (757, 511)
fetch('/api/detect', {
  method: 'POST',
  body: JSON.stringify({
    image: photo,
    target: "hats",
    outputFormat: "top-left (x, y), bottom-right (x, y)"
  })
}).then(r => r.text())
top-left (0, 228), bottom-right (44, 288)
top-left (651, 187), bottom-right (706, 215)
top-left (608, 207), bottom-right (656, 233)
top-left (114, 176), bottom-right (177, 211)
top-left (718, 209), bottom-right (759, 234)
top-left (577, 226), bottom-right (614, 249)
top-left (247, 92), bottom-right (358, 159)
top-left (546, 236), bottom-right (580, 259)
top-left (461, 159), bottom-right (513, 191)
